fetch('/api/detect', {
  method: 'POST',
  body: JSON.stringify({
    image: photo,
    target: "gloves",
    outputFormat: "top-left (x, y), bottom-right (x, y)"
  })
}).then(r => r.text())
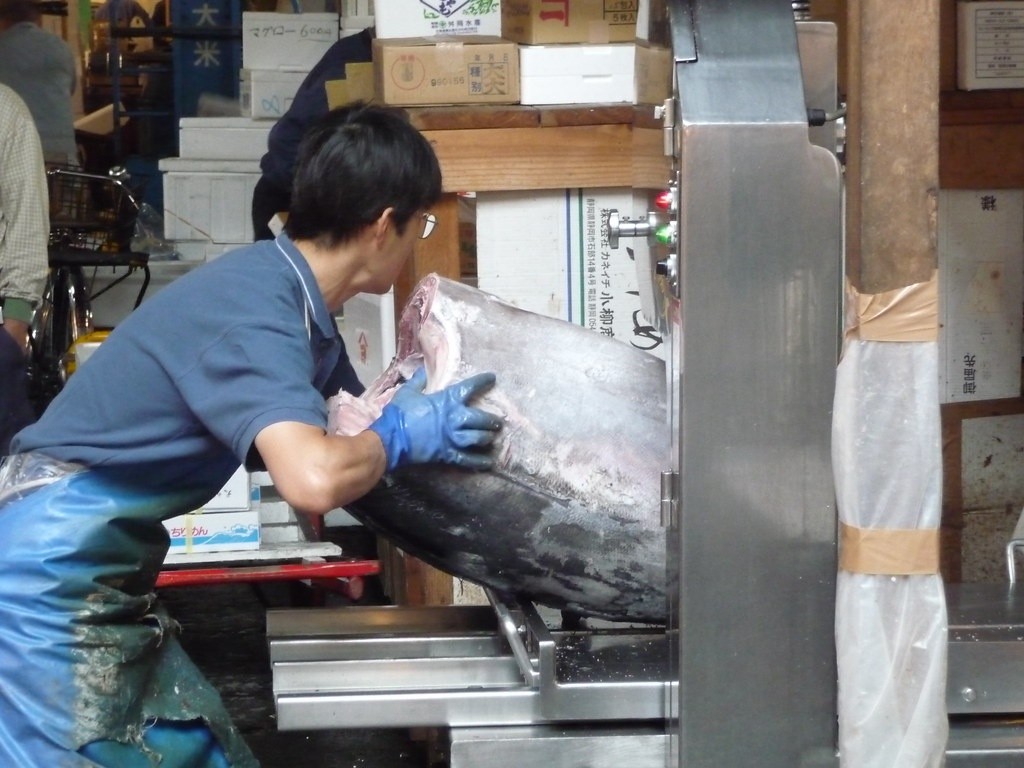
top-left (359, 365), bottom-right (502, 471)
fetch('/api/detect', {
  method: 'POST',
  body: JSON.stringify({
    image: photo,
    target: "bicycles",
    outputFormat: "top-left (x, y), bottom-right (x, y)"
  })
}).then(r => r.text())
top-left (30, 166), bottom-right (153, 400)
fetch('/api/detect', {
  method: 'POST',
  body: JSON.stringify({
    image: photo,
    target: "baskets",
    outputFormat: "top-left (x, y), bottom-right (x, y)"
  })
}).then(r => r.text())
top-left (42, 157), bottom-right (139, 252)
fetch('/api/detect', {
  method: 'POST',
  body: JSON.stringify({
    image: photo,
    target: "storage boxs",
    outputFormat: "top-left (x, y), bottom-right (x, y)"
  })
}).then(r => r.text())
top-left (160, 0), bottom-right (1021, 580)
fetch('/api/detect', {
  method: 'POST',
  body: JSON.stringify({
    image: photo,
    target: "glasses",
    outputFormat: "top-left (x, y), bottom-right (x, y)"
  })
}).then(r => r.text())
top-left (399, 208), bottom-right (437, 239)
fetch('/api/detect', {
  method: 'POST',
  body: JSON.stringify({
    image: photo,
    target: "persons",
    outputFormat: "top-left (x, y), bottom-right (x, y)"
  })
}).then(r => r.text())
top-left (0, 83), bottom-right (51, 458)
top-left (0, 100), bottom-right (504, 768)
top-left (251, 26), bottom-right (376, 242)
top-left (151, 0), bottom-right (166, 47)
top-left (0, 0), bottom-right (82, 197)
top-left (94, 0), bottom-right (151, 50)
